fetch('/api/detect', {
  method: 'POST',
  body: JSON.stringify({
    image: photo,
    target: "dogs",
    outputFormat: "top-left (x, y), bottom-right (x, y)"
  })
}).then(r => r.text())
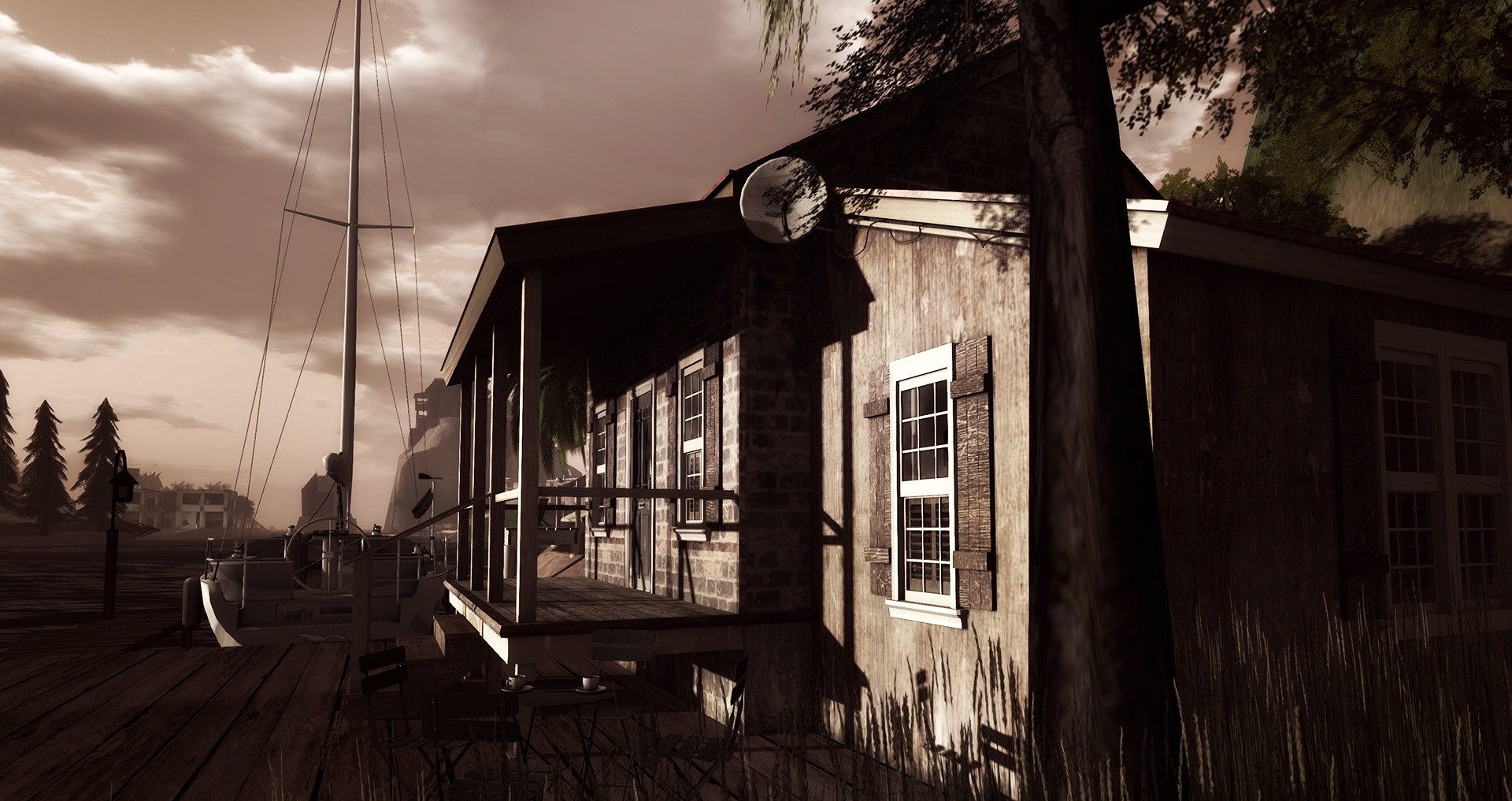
top-left (412, 542), bottom-right (424, 556)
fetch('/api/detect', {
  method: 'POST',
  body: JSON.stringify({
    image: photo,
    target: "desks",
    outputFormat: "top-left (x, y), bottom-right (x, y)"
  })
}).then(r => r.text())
top-left (495, 678), bottom-right (626, 801)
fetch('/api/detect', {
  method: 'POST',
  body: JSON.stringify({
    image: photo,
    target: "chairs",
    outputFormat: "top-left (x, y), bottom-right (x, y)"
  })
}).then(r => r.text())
top-left (360, 630), bottom-right (753, 801)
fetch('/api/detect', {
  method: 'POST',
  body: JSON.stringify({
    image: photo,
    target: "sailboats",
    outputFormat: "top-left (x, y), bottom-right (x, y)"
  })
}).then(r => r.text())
top-left (199, 0), bottom-right (456, 647)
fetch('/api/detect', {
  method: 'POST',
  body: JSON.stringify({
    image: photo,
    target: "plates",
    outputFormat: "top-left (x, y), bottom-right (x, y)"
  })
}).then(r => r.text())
top-left (501, 685), bottom-right (535, 692)
top-left (576, 685), bottom-right (607, 693)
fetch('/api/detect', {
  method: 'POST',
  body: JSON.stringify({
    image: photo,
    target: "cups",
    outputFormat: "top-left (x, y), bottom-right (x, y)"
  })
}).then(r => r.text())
top-left (582, 674), bottom-right (600, 691)
top-left (506, 674), bottom-right (526, 690)
